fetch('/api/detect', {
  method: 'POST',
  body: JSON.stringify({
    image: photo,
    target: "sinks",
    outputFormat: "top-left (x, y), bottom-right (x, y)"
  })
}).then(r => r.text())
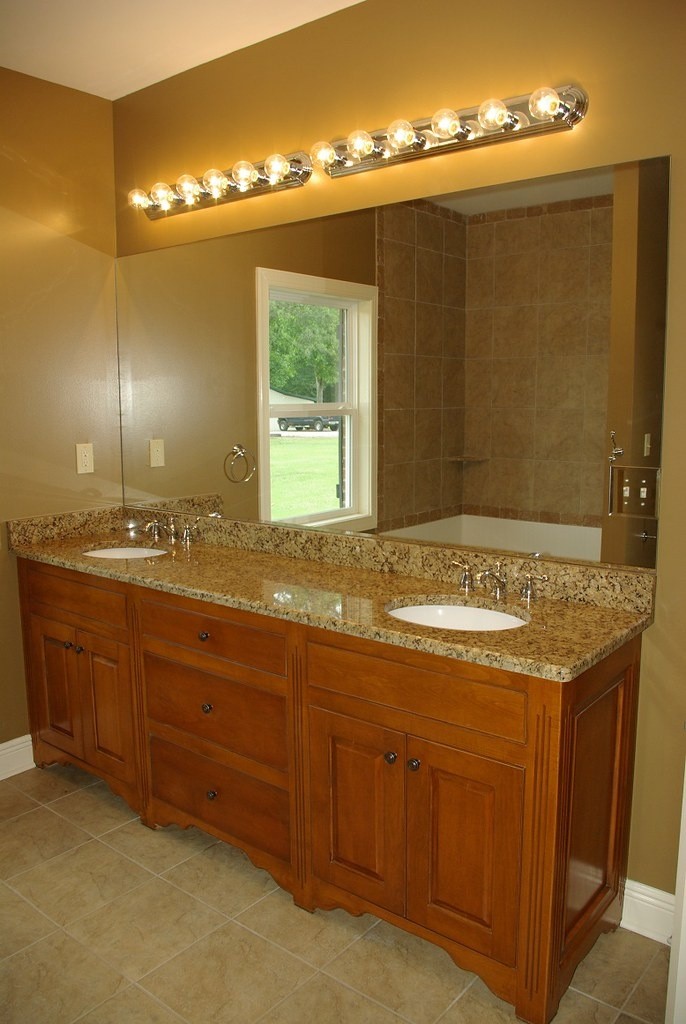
top-left (383, 595), bottom-right (531, 631)
top-left (79, 541), bottom-right (174, 558)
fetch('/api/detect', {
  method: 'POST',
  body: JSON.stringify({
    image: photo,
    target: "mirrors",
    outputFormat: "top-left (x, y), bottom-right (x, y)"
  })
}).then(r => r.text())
top-left (113, 155), bottom-right (670, 566)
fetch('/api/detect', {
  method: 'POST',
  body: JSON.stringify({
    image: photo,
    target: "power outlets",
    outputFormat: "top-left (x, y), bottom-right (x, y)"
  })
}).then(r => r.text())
top-left (75, 443), bottom-right (94, 474)
top-left (149, 440), bottom-right (165, 468)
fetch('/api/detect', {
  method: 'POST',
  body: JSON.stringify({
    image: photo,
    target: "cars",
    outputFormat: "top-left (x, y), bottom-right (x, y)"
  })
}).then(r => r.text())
top-left (278, 416), bottom-right (339, 431)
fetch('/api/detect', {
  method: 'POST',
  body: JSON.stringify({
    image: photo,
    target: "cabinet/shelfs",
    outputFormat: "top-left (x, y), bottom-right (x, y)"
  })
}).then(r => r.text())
top-left (17, 556), bottom-right (642, 1024)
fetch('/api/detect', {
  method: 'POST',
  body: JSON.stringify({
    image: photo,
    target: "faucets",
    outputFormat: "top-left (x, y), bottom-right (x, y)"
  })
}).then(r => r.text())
top-left (153, 517), bottom-right (178, 544)
top-left (471, 571), bottom-right (506, 597)
